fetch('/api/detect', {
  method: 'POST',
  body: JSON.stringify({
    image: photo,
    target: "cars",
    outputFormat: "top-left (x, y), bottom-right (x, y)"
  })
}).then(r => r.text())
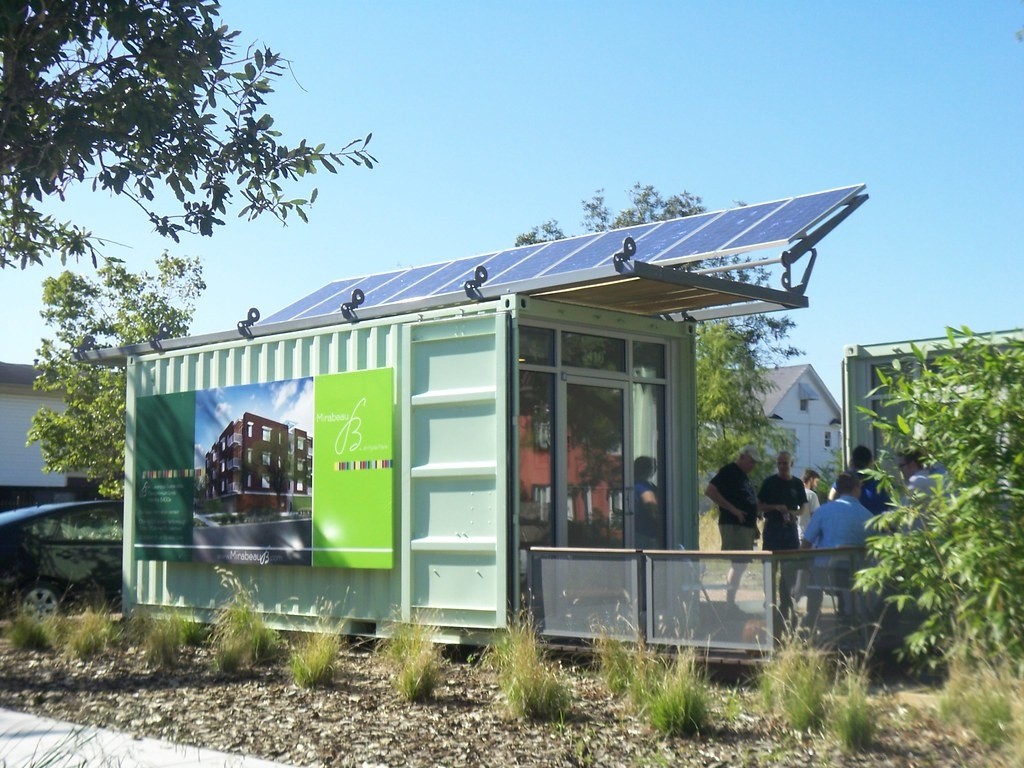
top-left (0, 501), bottom-right (121, 627)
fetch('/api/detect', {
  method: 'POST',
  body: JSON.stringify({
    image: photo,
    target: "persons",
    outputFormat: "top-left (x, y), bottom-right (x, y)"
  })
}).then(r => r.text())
top-left (796, 466), bottom-right (821, 547)
top-left (797, 444), bottom-right (894, 631)
top-left (704, 445), bottom-right (763, 618)
top-left (634, 454), bottom-right (659, 551)
top-left (897, 448), bottom-right (936, 534)
top-left (755, 449), bottom-right (809, 618)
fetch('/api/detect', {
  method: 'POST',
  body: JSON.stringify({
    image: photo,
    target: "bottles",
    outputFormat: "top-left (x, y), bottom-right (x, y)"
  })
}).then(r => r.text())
top-left (783, 512), bottom-right (791, 527)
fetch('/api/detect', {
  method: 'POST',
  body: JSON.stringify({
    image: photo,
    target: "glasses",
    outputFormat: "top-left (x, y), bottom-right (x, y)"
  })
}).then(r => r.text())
top-left (898, 464), bottom-right (906, 470)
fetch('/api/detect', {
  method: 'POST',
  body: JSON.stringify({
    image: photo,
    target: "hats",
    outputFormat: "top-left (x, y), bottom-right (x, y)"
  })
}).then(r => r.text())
top-left (739, 444), bottom-right (764, 463)
